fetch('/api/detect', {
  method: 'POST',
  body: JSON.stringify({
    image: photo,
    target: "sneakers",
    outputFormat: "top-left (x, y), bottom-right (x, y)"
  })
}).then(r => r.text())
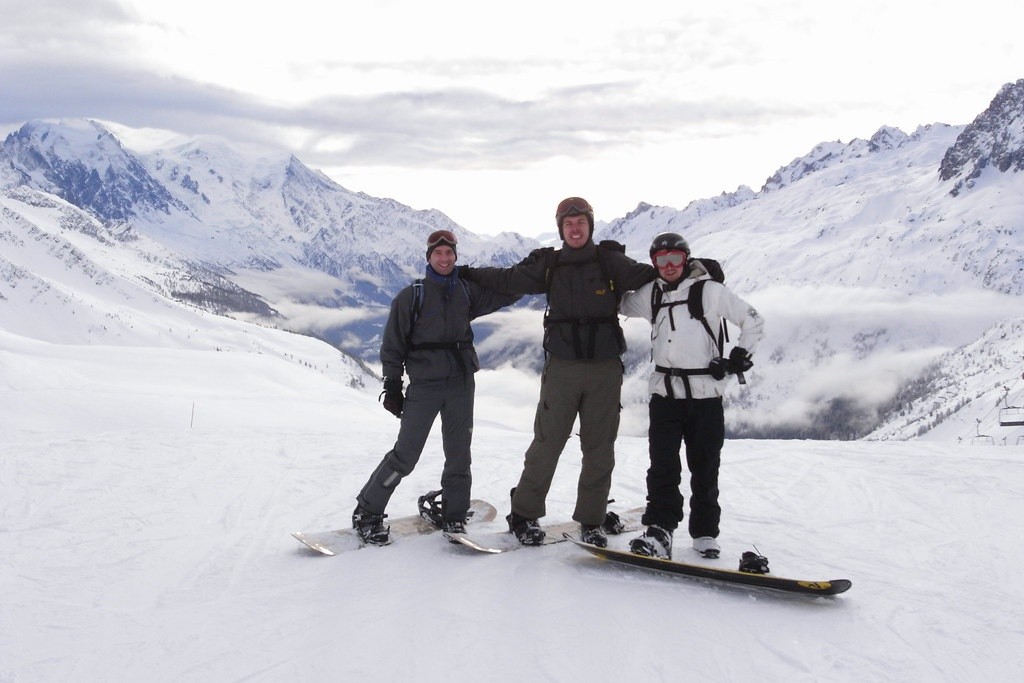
top-left (630, 525), bottom-right (672, 560)
top-left (692, 536), bottom-right (721, 558)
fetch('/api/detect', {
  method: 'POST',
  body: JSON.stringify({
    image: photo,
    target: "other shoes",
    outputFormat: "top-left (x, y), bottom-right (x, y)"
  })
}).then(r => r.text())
top-left (506, 513), bottom-right (545, 545)
top-left (581, 524), bottom-right (607, 547)
top-left (353, 511), bottom-right (388, 545)
top-left (443, 520), bottom-right (466, 544)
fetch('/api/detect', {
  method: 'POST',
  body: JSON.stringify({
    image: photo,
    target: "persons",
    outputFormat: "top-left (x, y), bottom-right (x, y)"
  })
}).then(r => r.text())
top-left (456, 198), bottom-right (725, 549)
top-left (352, 230), bottom-right (553, 547)
top-left (619, 234), bottom-right (764, 557)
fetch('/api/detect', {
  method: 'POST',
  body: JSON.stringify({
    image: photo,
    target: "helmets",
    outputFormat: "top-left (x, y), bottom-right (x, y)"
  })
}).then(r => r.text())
top-left (418, 489), bottom-right (473, 528)
top-left (426, 230), bottom-right (457, 256)
top-left (650, 232), bottom-right (690, 258)
top-left (556, 197), bottom-right (595, 241)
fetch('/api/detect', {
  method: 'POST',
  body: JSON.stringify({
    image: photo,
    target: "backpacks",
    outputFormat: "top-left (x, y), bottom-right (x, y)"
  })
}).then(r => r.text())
top-left (682, 257), bottom-right (723, 357)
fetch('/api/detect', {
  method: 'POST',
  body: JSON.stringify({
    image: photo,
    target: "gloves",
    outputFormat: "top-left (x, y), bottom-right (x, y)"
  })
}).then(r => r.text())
top-left (518, 248), bottom-right (555, 265)
top-left (599, 240), bottom-right (626, 256)
top-left (453, 265), bottom-right (470, 279)
top-left (709, 346), bottom-right (753, 380)
top-left (383, 376), bottom-right (404, 418)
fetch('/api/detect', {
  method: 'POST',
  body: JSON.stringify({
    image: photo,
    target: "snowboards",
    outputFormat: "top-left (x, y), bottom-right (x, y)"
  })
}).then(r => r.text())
top-left (561, 532), bottom-right (853, 596)
top-left (290, 498), bottom-right (498, 557)
top-left (443, 505), bottom-right (651, 554)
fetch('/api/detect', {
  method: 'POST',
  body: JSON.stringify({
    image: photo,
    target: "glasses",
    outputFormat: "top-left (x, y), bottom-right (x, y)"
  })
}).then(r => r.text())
top-left (653, 251), bottom-right (686, 269)
top-left (556, 197), bottom-right (593, 216)
top-left (426, 230), bottom-right (457, 246)
